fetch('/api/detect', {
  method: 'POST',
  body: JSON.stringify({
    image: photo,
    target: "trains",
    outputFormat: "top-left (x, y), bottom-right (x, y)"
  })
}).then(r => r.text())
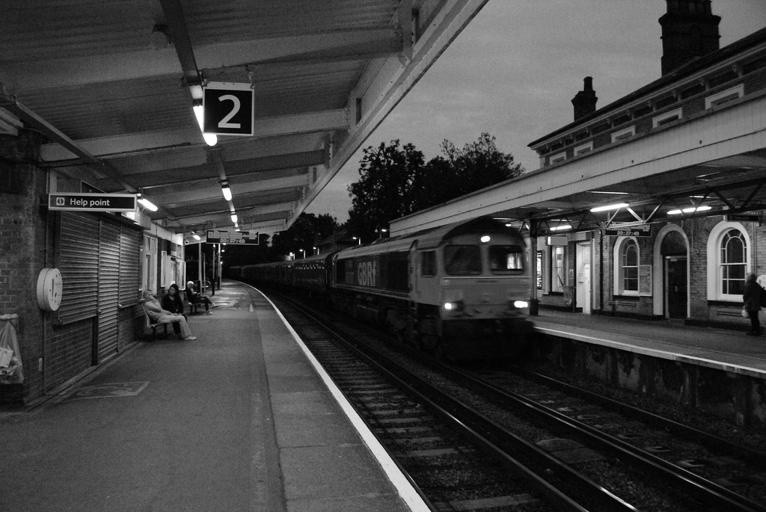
top-left (205, 214), bottom-right (534, 351)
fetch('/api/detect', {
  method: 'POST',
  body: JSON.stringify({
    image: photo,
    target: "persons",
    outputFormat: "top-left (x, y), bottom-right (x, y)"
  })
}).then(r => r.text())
top-left (743, 273), bottom-right (766, 336)
top-left (161, 284), bottom-right (187, 334)
top-left (186, 281), bottom-right (219, 315)
top-left (143, 289), bottom-right (197, 341)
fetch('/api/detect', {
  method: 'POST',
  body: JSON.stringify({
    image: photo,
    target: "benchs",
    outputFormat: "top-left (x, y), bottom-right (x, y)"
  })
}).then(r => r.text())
top-left (183, 290), bottom-right (202, 314)
top-left (139, 302), bottom-right (169, 342)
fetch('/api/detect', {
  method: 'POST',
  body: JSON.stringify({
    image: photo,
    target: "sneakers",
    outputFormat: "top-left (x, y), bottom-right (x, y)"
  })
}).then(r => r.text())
top-left (211, 303), bottom-right (218, 309)
top-left (184, 336), bottom-right (196, 341)
top-left (206, 310), bottom-right (213, 315)
